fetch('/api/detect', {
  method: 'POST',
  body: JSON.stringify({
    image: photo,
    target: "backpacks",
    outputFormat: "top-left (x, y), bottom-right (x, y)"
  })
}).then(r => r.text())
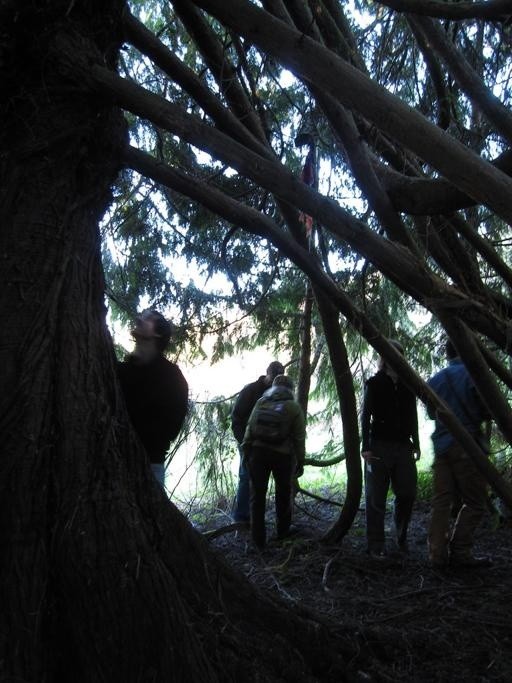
top-left (252, 398), bottom-right (297, 443)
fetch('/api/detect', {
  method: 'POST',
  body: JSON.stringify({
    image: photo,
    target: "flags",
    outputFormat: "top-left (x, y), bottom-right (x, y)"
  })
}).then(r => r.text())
top-left (298, 151), bottom-right (316, 238)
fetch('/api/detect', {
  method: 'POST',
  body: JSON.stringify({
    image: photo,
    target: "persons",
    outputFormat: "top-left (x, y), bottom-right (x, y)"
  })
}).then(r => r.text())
top-left (241, 374), bottom-right (307, 547)
top-left (231, 361), bottom-right (285, 524)
top-left (361, 338), bottom-right (421, 559)
top-left (427, 340), bottom-right (492, 572)
top-left (119, 307), bottom-right (189, 488)
